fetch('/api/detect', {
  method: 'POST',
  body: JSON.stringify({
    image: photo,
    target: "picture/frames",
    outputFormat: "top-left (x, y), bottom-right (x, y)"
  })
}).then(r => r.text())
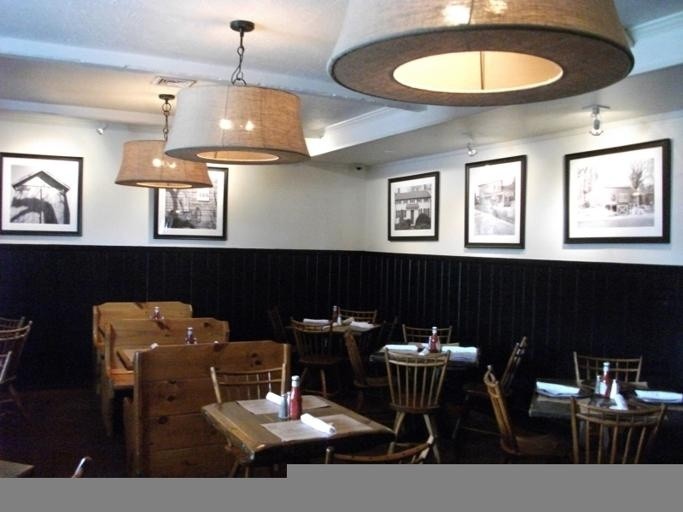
top-left (153, 166), bottom-right (229, 241)
top-left (464, 154), bottom-right (528, 250)
top-left (387, 171), bottom-right (440, 242)
top-left (562, 138), bottom-right (672, 245)
top-left (0, 152), bottom-right (84, 237)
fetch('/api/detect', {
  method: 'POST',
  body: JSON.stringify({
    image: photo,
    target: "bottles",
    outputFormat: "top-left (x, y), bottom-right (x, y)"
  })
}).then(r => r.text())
top-left (600, 362), bottom-right (611, 397)
top-left (332, 306), bottom-right (337, 321)
top-left (289, 375), bottom-right (301, 418)
top-left (186, 327), bottom-right (193, 344)
top-left (153, 306), bottom-right (162, 320)
top-left (430, 326), bottom-right (438, 352)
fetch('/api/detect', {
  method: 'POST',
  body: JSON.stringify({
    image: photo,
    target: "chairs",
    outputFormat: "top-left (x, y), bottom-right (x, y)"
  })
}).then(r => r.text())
top-left (0, 302), bottom-right (683, 478)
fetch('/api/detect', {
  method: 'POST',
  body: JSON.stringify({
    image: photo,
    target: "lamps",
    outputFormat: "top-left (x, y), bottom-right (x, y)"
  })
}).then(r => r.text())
top-left (583, 104), bottom-right (610, 136)
top-left (96, 122), bottom-right (108, 135)
top-left (466, 142), bottom-right (478, 157)
top-left (326, 0), bottom-right (635, 108)
top-left (114, 93), bottom-right (213, 189)
top-left (163, 20), bottom-right (312, 165)
